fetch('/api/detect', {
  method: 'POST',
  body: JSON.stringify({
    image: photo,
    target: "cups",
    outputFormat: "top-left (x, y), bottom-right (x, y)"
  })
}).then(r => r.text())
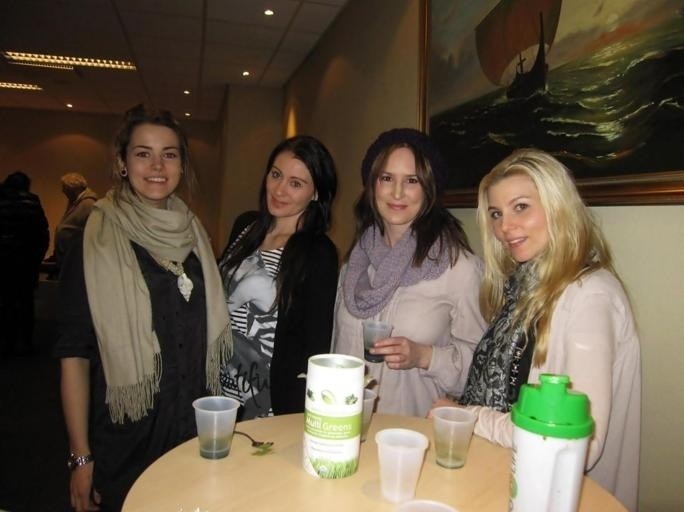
top-left (361, 321), bottom-right (395, 363)
top-left (374, 427), bottom-right (430, 502)
top-left (300, 354), bottom-right (367, 482)
top-left (430, 405), bottom-right (477, 470)
top-left (191, 395), bottom-right (242, 460)
top-left (360, 387), bottom-right (379, 442)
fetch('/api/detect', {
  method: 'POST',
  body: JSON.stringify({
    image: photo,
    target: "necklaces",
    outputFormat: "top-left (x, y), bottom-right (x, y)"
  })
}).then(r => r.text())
top-left (154, 255), bottom-right (193, 302)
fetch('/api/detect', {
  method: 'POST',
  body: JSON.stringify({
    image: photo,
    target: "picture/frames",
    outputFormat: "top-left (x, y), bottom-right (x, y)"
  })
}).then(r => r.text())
top-left (416, 1), bottom-right (684, 209)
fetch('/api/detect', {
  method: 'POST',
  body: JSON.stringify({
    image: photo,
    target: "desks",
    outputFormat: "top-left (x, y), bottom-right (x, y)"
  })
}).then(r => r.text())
top-left (121, 412), bottom-right (627, 512)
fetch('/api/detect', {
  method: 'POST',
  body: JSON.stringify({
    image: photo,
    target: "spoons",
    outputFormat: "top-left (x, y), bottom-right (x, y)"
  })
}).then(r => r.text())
top-left (233, 430), bottom-right (274, 449)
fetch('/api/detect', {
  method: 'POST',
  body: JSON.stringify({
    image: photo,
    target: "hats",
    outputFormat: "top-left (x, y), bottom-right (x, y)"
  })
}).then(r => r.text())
top-left (361, 128), bottom-right (448, 194)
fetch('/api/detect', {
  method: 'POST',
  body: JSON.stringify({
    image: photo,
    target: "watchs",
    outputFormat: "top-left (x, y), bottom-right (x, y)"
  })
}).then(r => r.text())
top-left (64, 447), bottom-right (94, 471)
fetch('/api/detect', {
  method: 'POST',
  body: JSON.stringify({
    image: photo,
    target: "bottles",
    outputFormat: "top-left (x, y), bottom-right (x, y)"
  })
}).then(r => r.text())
top-left (510, 373), bottom-right (596, 512)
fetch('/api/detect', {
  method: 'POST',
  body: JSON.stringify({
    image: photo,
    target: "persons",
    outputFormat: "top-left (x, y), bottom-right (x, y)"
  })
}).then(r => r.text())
top-left (222, 251), bottom-right (279, 422)
top-left (213, 133), bottom-right (341, 423)
top-left (329, 127), bottom-right (490, 418)
top-left (43, 170), bottom-right (100, 281)
top-left (0, 170), bottom-right (50, 356)
top-left (46, 103), bottom-right (234, 511)
top-left (425, 145), bottom-right (642, 511)
